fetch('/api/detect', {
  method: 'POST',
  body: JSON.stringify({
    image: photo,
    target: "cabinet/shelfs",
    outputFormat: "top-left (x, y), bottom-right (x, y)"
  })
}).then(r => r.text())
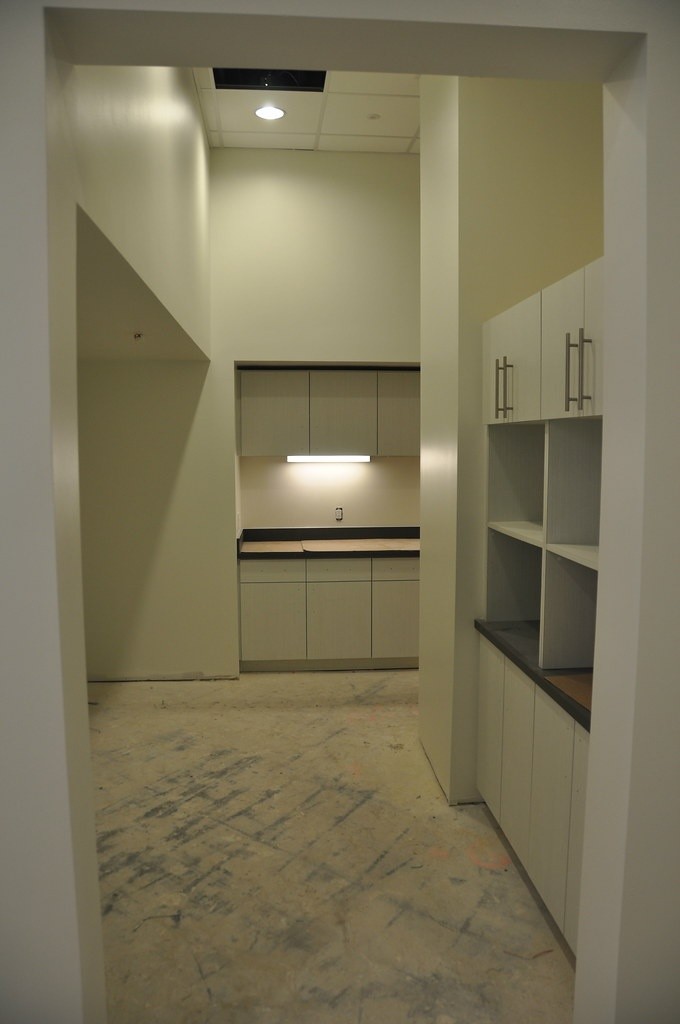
top-left (235, 365), bottom-right (417, 676)
top-left (472, 251), bottom-right (607, 972)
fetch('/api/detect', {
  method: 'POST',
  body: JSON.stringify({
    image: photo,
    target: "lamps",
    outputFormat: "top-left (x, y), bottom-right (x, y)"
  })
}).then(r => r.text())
top-left (285, 456), bottom-right (371, 463)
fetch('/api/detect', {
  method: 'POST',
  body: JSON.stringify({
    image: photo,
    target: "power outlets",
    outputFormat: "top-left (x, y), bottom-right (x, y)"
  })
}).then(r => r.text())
top-left (335, 508), bottom-right (343, 520)
